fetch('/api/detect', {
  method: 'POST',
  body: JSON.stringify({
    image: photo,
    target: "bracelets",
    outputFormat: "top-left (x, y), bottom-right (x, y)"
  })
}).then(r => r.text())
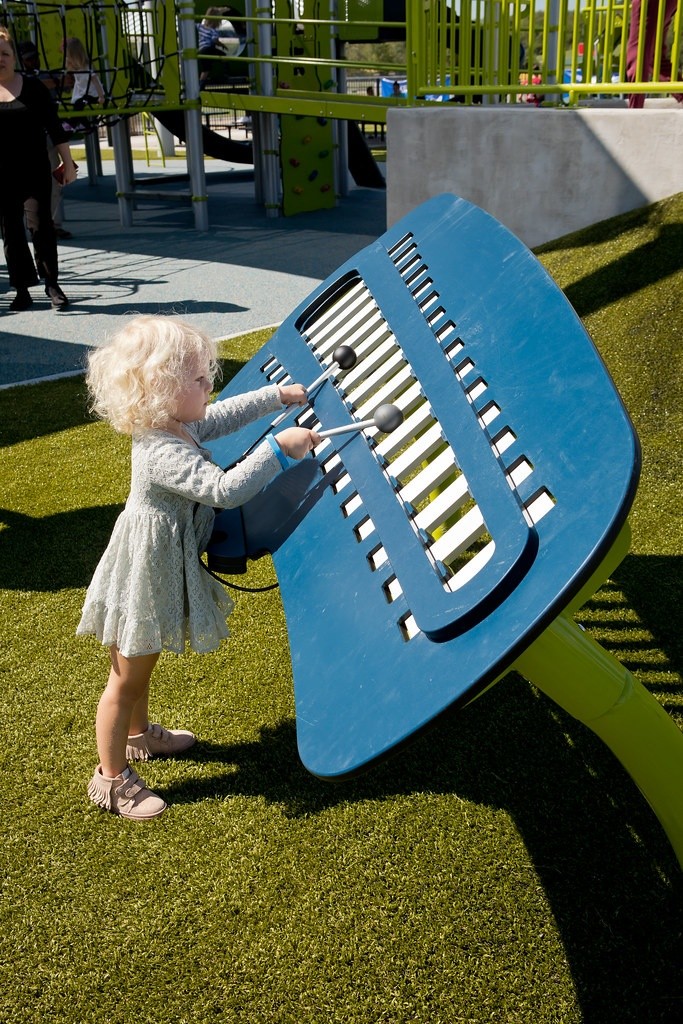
top-left (265, 434), bottom-right (290, 472)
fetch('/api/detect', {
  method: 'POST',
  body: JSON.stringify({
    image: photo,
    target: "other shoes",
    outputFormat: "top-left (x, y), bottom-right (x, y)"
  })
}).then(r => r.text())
top-left (8, 291), bottom-right (33, 309)
top-left (56, 228), bottom-right (74, 240)
top-left (44, 283), bottom-right (70, 308)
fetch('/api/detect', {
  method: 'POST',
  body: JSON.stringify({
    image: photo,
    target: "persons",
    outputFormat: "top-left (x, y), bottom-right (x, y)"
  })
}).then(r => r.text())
top-left (367, 63), bottom-right (544, 105)
top-left (0, 26), bottom-right (77, 311)
top-left (195, 6), bottom-right (228, 88)
top-left (16, 38), bottom-right (107, 241)
top-left (76, 312), bottom-right (322, 820)
top-left (625, 0), bottom-right (683, 109)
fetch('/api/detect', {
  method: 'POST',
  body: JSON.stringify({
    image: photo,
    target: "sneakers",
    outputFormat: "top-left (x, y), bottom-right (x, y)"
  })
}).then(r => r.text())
top-left (125, 723), bottom-right (196, 760)
top-left (87, 762), bottom-right (167, 820)
